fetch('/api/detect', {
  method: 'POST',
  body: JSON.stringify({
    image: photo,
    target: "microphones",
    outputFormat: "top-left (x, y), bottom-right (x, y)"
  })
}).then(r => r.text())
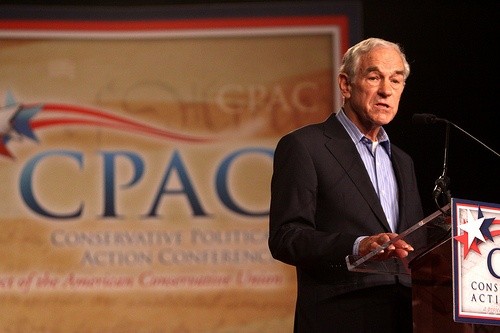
top-left (414, 113), bottom-right (500, 198)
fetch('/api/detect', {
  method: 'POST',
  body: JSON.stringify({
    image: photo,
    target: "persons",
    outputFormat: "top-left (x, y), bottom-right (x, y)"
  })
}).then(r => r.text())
top-left (268, 38), bottom-right (424, 333)
